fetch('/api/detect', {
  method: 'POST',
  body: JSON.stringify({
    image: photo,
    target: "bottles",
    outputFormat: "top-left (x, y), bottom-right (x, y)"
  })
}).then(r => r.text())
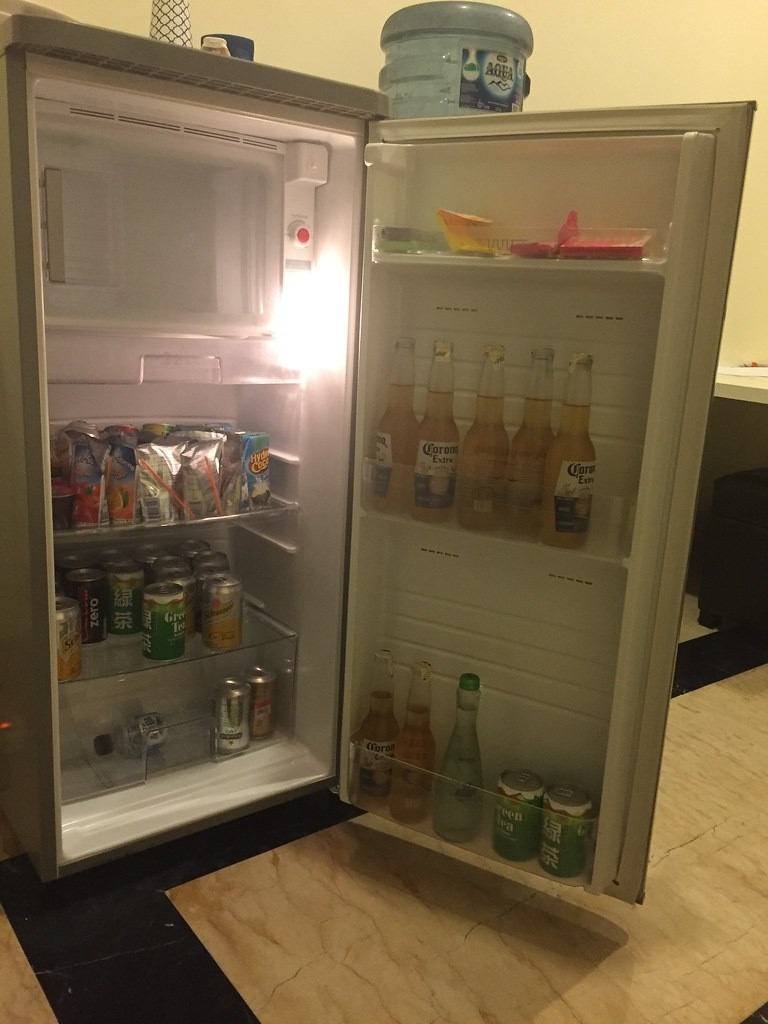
top-left (430, 672), bottom-right (483, 842)
top-left (410, 341), bottom-right (460, 522)
top-left (377, 1), bottom-right (533, 121)
top-left (504, 347), bottom-right (554, 533)
top-left (346, 649), bottom-right (394, 811)
top-left (370, 338), bottom-right (412, 514)
top-left (456, 345), bottom-right (509, 531)
top-left (543, 354), bottom-right (597, 546)
top-left (391, 661), bottom-right (431, 823)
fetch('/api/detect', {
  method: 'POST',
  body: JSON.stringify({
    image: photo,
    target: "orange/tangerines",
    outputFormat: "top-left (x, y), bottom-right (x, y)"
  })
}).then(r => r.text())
top-left (106, 486), bottom-right (128, 512)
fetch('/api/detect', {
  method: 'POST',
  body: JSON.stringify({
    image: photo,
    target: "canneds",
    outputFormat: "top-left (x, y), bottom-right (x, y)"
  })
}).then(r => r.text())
top-left (538, 785), bottom-right (594, 878)
top-left (63, 420), bottom-right (175, 446)
top-left (492, 769), bottom-right (545, 862)
top-left (55, 537), bottom-right (244, 683)
top-left (210, 665), bottom-right (277, 757)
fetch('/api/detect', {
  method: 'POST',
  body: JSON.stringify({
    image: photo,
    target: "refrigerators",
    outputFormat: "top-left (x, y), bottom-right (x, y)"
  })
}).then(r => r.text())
top-left (6, 10), bottom-right (758, 904)
